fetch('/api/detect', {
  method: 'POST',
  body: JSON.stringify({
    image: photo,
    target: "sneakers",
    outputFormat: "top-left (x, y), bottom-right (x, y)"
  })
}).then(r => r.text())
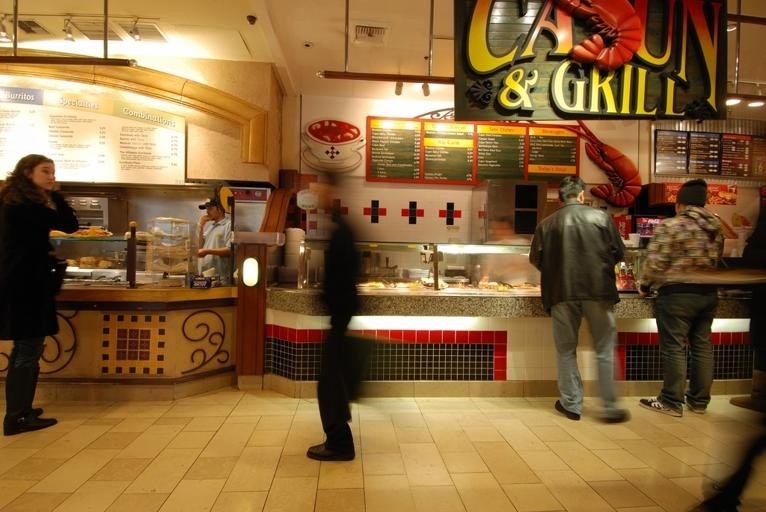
top-left (601, 408), bottom-right (631, 423)
top-left (554, 399), bottom-right (580, 421)
top-left (639, 397), bottom-right (683, 417)
top-left (684, 395), bottom-right (705, 414)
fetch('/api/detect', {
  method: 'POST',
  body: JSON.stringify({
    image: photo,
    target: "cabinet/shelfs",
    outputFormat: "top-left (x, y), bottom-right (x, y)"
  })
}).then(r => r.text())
top-left (291, 237), bottom-right (541, 297)
top-left (50, 235), bottom-right (196, 287)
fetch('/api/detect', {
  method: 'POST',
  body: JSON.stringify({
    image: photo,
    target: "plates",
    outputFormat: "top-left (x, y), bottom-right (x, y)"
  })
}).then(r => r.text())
top-left (300, 147), bottom-right (364, 173)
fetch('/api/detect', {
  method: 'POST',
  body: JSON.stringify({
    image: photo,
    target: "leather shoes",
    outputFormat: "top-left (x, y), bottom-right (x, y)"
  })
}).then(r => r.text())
top-left (30, 408), bottom-right (43, 417)
top-left (307, 440), bottom-right (356, 461)
top-left (3, 414), bottom-right (58, 436)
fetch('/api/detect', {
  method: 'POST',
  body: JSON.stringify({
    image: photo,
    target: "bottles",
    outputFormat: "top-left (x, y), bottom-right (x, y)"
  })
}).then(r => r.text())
top-left (615, 262), bottom-right (635, 290)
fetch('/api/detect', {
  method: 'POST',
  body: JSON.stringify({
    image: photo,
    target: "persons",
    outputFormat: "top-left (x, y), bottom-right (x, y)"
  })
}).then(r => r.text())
top-left (636, 176), bottom-right (726, 419)
top-left (528, 173), bottom-right (631, 425)
top-left (0, 153), bottom-right (80, 439)
top-left (730, 194), bottom-right (765, 414)
top-left (478, 214), bottom-right (532, 291)
top-left (195, 197), bottom-right (236, 283)
top-left (303, 181), bottom-right (366, 464)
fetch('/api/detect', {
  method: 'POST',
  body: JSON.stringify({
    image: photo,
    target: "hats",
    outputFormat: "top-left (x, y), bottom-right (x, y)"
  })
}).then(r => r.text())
top-left (198, 196), bottom-right (219, 210)
top-left (675, 178), bottom-right (708, 206)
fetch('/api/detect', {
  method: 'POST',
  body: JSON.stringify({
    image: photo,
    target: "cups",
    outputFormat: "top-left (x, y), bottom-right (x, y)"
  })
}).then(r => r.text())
top-left (300, 119), bottom-right (367, 163)
top-left (629, 233), bottom-right (641, 247)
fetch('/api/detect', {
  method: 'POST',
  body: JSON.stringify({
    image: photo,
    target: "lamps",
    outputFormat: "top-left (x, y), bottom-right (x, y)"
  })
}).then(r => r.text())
top-left (394, 75), bottom-right (431, 96)
top-left (726, 94), bottom-right (766, 114)
top-left (1, 10), bottom-right (159, 40)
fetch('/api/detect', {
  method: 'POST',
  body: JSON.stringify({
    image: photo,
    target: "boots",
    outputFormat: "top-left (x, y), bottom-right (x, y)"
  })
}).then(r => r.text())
top-left (729, 369), bottom-right (766, 412)
